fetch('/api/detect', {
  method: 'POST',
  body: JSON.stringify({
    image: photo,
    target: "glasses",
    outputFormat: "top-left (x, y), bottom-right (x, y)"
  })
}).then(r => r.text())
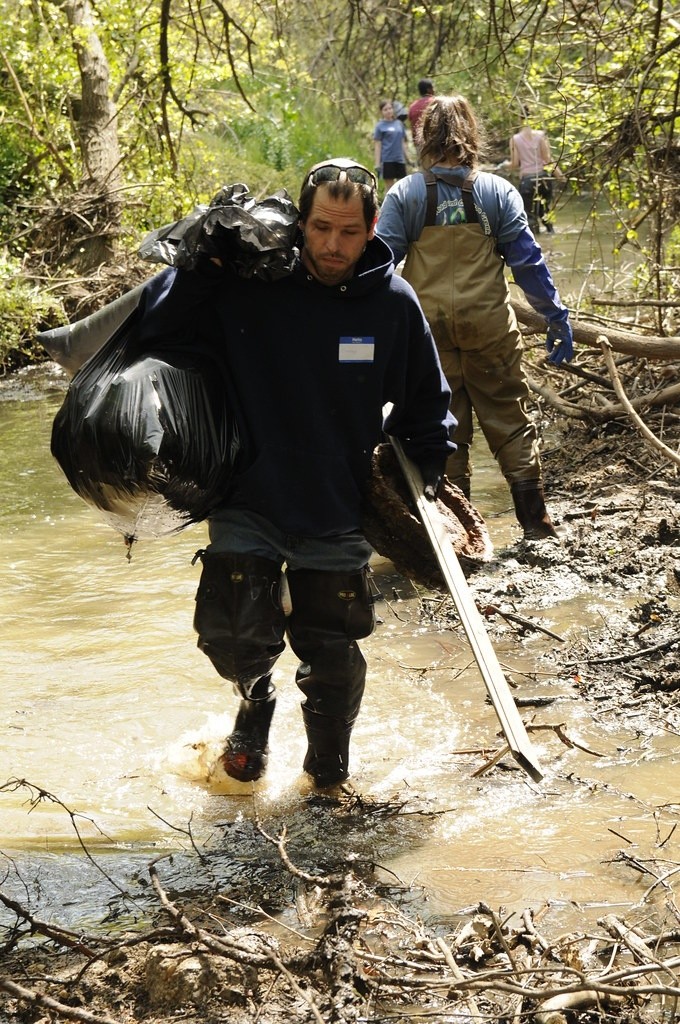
top-left (301, 164), bottom-right (377, 192)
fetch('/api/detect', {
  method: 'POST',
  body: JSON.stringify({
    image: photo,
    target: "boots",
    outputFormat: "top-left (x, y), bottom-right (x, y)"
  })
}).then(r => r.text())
top-left (299, 699), bottom-right (364, 787)
top-left (509, 478), bottom-right (561, 540)
top-left (449, 477), bottom-right (471, 503)
top-left (194, 640), bottom-right (287, 783)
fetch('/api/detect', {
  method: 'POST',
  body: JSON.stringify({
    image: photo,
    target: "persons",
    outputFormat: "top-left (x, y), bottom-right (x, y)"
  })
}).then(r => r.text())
top-left (372, 96), bottom-right (574, 541)
top-left (373, 100), bottom-right (409, 193)
top-left (509, 103), bottom-right (555, 234)
top-left (131, 159), bottom-right (459, 789)
top-left (407, 78), bottom-right (435, 173)
top-left (391, 100), bottom-right (408, 121)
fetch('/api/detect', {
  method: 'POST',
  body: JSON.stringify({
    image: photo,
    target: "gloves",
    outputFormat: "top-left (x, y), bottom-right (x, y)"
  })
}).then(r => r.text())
top-left (495, 225), bottom-right (574, 366)
